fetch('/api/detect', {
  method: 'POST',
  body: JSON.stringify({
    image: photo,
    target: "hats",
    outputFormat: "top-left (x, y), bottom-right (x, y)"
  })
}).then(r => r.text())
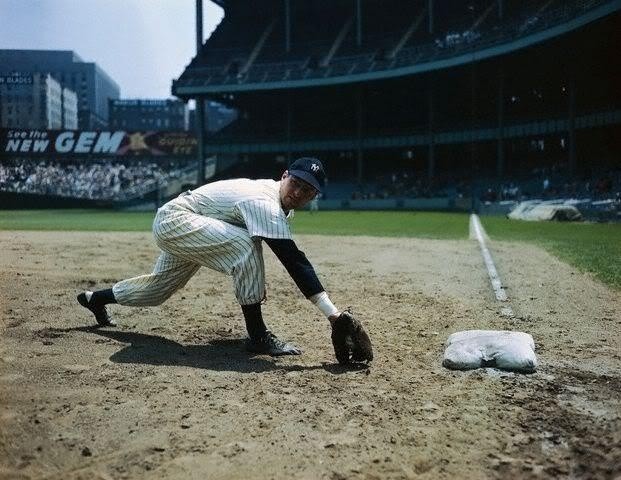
top-left (288, 157), bottom-right (326, 194)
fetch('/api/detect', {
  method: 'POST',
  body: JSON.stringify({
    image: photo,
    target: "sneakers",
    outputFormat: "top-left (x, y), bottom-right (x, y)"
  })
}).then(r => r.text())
top-left (77, 291), bottom-right (117, 327)
top-left (246, 331), bottom-right (304, 355)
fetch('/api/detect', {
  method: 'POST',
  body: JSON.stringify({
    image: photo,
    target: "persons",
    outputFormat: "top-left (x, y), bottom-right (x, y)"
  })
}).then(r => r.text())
top-left (310, 193), bottom-right (322, 213)
top-left (0, 157), bottom-right (188, 203)
top-left (435, 27), bottom-right (481, 51)
top-left (76, 156), bottom-right (376, 368)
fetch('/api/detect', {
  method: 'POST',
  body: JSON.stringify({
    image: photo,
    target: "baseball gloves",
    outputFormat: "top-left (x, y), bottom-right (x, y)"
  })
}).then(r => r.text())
top-left (329, 310), bottom-right (374, 370)
